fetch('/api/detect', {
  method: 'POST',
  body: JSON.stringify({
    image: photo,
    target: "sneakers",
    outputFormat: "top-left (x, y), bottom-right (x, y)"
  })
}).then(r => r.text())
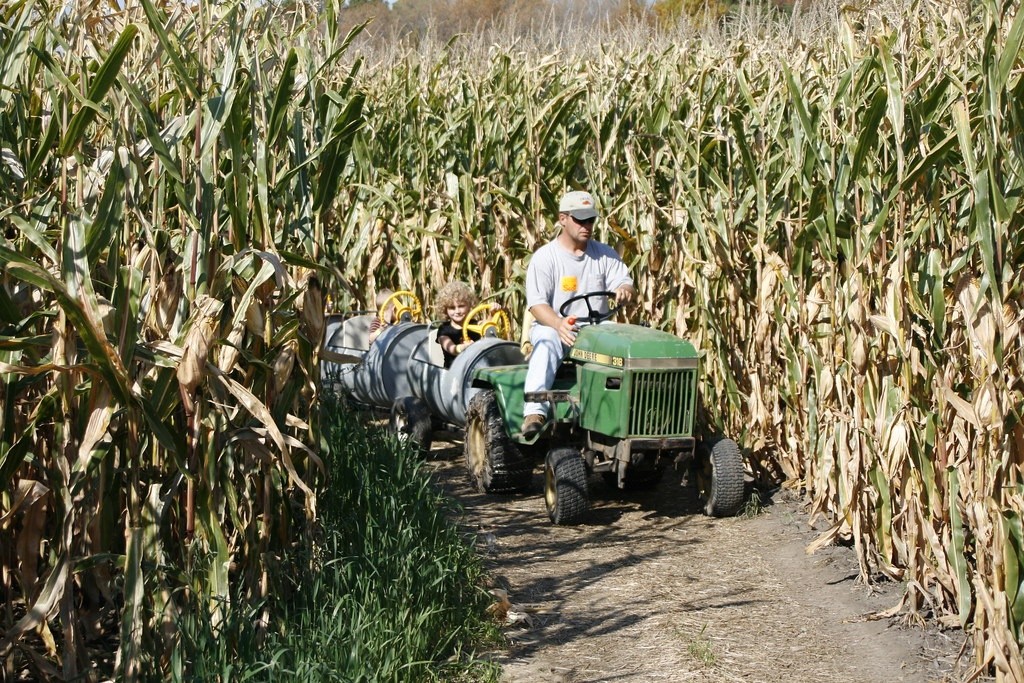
top-left (521, 414), bottom-right (544, 436)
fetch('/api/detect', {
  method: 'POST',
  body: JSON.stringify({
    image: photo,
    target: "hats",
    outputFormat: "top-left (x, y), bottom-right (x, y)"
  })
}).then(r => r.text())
top-left (559, 190), bottom-right (602, 220)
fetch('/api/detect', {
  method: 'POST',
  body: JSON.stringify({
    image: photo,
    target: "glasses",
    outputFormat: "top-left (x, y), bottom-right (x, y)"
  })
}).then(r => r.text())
top-left (570, 215), bottom-right (595, 224)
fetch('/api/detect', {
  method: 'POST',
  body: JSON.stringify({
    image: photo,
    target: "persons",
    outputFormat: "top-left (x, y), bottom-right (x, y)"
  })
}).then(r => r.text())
top-left (369, 289), bottom-right (402, 351)
top-left (435, 282), bottom-right (502, 371)
top-left (521, 190), bottom-right (637, 437)
